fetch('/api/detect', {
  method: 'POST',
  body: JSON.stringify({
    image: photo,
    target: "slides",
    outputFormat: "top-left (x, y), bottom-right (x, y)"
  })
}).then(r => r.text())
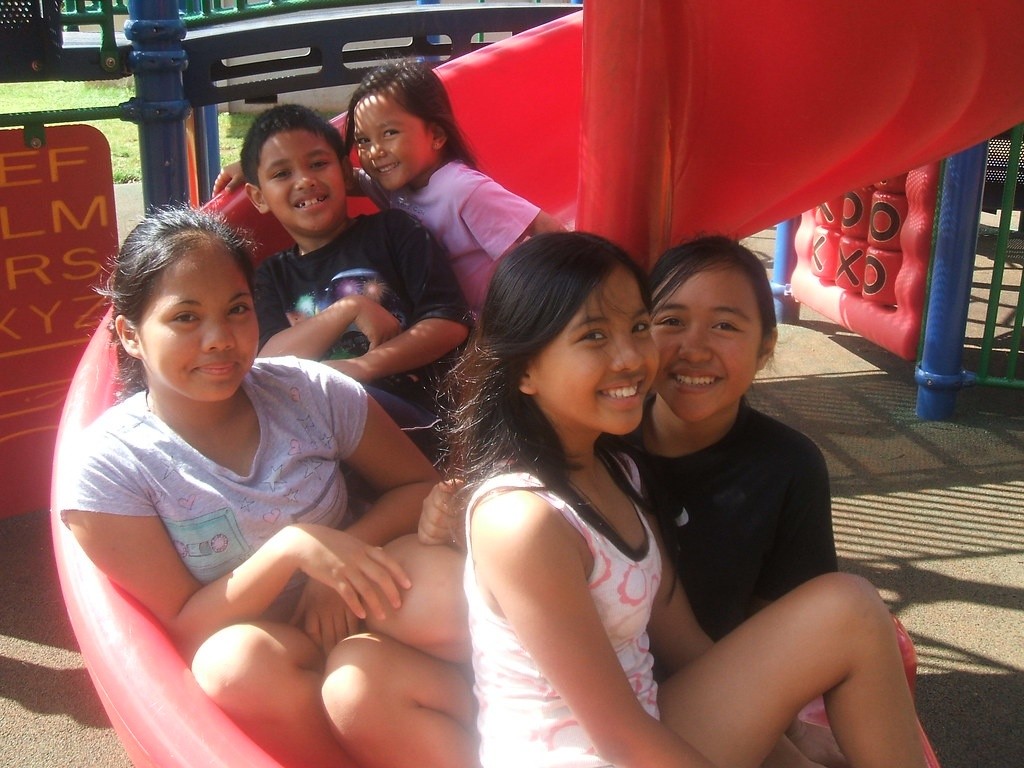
top-left (51, 0), bottom-right (1024, 768)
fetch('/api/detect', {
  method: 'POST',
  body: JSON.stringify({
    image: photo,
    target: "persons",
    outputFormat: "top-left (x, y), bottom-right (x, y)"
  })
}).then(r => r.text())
top-left (210, 59), bottom-right (572, 353)
top-left (56, 209), bottom-right (477, 768)
top-left (462, 232), bottom-right (932, 768)
top-left (232, 101), bottom-right (468, 467)
top-left (413, 236), bottom-right (840, 645)
